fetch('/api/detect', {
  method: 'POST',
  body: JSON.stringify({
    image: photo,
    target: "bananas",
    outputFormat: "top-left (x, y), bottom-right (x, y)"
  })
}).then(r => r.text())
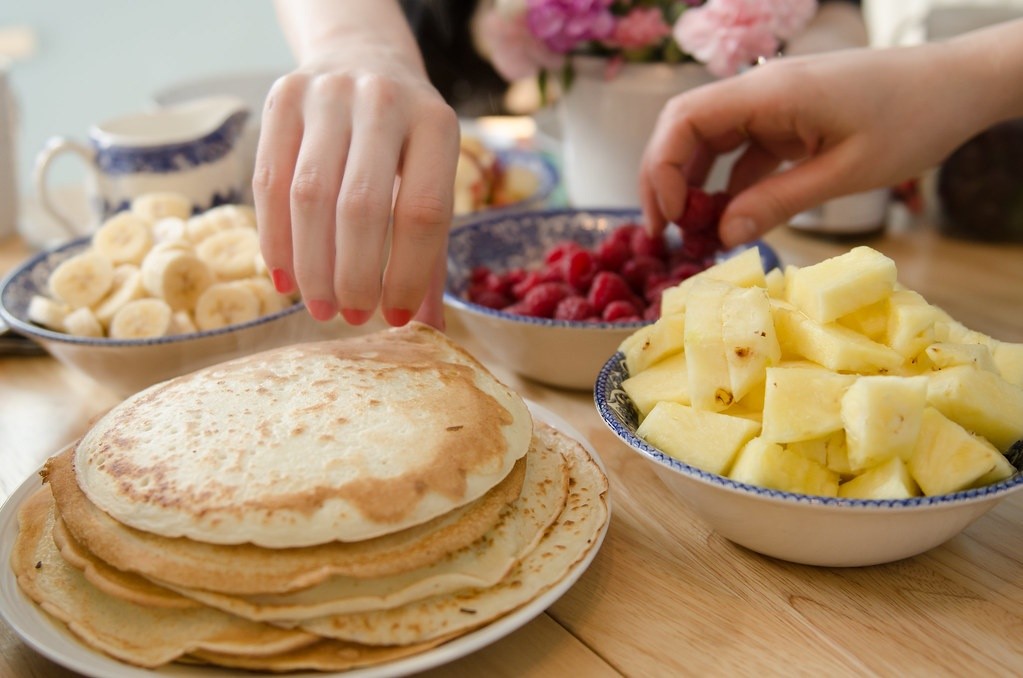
top-left (27, 193), bottom-right (304, 343)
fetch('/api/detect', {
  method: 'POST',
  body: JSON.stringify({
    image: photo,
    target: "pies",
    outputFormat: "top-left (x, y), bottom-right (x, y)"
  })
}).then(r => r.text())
top-left (11, 321), bottom-right (609, 671)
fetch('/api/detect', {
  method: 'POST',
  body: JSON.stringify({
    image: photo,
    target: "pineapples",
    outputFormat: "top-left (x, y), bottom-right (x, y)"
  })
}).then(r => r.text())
top-left (613, 246), bottom-right (1023, 502)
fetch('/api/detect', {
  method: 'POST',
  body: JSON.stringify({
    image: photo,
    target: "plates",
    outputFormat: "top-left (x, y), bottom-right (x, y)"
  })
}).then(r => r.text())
top-left (0, 397), bottom-right (612, 677)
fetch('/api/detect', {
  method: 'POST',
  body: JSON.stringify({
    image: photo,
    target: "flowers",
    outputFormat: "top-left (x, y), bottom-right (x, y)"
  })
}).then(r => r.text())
top-left (521, 0), bottom-right (823, 107)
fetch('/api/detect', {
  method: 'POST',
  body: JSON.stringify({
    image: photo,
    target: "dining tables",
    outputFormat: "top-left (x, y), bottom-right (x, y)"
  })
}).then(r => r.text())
top-left (3, 200), bottom-right (1022, 677)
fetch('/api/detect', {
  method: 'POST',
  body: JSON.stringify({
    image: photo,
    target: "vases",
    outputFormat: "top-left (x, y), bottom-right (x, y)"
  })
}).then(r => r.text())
top-left (553, 56), bottom-right (749, 210)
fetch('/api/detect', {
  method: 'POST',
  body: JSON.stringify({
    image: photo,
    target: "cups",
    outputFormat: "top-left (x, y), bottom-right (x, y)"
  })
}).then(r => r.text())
top-left (36, 98), bottom-right (247, 233)
top-left (450, 115), bottom-right (551, 216)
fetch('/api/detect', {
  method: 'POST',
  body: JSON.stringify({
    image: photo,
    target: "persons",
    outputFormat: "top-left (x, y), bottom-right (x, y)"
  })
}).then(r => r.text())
top-left (645, 18), bottom-right (1023, 248)
top-left (253, 0), bottom-right (869, 325)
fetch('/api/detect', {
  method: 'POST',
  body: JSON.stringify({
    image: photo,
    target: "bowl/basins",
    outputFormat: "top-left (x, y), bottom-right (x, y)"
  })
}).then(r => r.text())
top-left (442, 203), bottom-right (782, 391)
top-left (1, 235), bottom-right (325, 394)
top-left (594, 347), bottom-right (1023, 563)
